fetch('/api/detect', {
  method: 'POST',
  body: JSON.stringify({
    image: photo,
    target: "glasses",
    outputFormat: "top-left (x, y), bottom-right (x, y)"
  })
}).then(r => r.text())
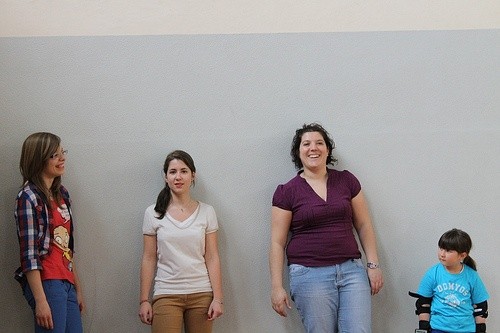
top-left (49, 149), bottom-right (68, 159)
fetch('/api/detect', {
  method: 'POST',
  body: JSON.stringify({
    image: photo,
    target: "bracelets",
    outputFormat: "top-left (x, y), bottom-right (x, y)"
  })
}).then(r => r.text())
top-left (140, 299), bottom-right (150, 305)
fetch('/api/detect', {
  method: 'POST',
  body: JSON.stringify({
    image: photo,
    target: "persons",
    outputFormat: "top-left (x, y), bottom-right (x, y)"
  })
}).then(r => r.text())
top-left (15, 132), bottom-right (84, 333)
top-left (409, 229), bottom-right (490, 333)
top-left (271, 122), bottom-right (384, 333)
top-left (139, 150), bottom-right (224, 333)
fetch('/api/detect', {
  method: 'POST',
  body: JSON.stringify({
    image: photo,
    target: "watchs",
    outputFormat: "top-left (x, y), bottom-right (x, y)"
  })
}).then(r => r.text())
top-left (367, 263), bottom-right (380, 268)
